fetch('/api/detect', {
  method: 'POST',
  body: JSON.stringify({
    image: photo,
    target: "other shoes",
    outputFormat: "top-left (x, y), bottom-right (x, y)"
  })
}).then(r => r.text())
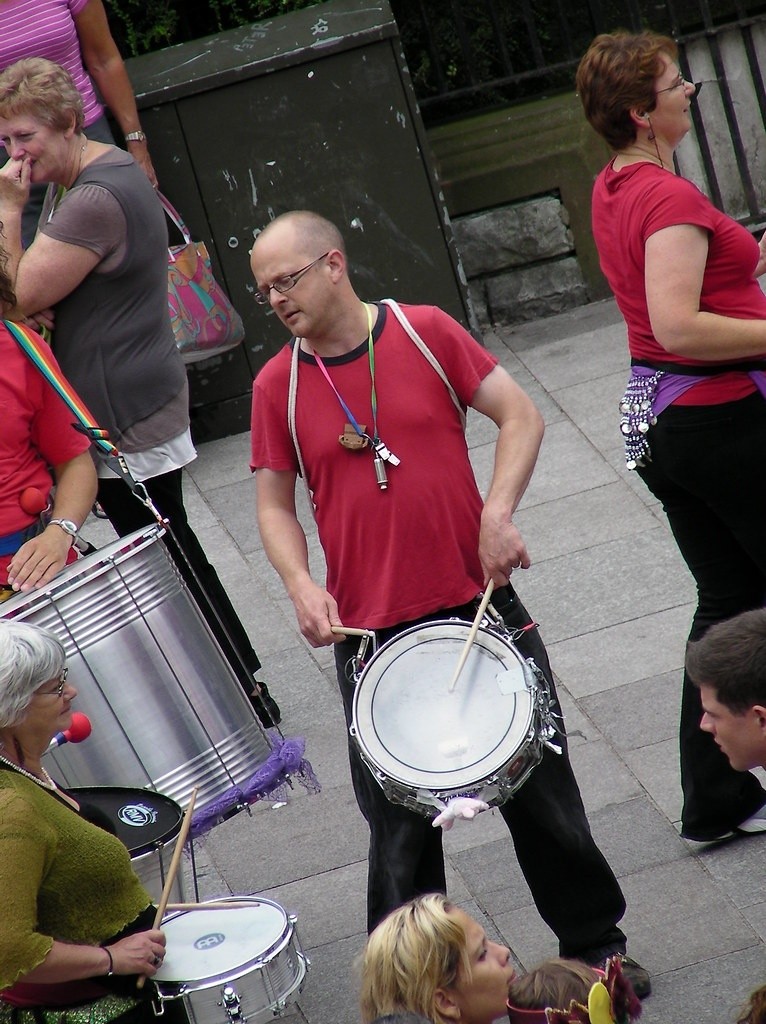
top-left (248, 681), bottom-right (281, 727)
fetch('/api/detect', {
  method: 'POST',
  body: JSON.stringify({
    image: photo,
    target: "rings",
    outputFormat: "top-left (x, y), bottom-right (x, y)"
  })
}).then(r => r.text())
top-left (151, 181), bottom-right (156, 188)
top-left (150, 955), bottom-right (160, 966)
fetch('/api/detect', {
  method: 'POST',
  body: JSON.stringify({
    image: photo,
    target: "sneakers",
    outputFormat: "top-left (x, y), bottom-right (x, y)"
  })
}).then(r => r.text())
top-left (591, 952), bottom-right (652, 996)
top-left (682, 803), bottom-right (766, 841)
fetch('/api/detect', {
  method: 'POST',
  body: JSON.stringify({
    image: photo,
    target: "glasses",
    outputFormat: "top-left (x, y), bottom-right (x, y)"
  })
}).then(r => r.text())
top-left (655, 75), bottom-right (687, 94)
top-left (35, 668), bottom-right (69, 697)
top-left (253, 252), bottom-right (330, 305)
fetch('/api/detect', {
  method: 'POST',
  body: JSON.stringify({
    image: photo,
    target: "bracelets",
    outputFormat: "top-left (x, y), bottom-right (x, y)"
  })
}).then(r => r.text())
top-left (101, 946), bottom-right (114, 977)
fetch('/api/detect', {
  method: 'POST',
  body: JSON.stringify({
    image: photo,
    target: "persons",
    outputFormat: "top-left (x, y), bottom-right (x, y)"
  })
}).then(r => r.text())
top-left (353, 892), bottom-right (518, 1024)
top-left (578, 31), bottom-right (766, 841)
top-left (0, 58), bottom-right (283, 729)
top-left (0, 0), bottom-right (158, 253)
top-left (251, 210), bottom-right (653, 1000)
top-left (0, 621), bottom-right (167, 1024)
top-left (0, 222), bottom-right (98, 615)
top-left (507, 951), bottom-right (643, 1024)
top-left (687, 605), bottom-right (766, 772)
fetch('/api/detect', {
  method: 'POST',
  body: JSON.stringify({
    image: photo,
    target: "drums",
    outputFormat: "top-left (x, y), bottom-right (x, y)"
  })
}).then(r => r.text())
top-left (0, 521), bottom-right (305, 842)
top-left (146, 895), bottom-right (308, 1024)
top-left (60, 784), bottom-right (189, 922)
top-left (351, 617), bottom-right (546, 819)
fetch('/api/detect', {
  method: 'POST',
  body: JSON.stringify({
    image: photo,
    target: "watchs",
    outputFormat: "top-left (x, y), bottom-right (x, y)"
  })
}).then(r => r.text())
top-left (124, 131), bottom-right (147, 141)
top-left (47, 519), bottom-right (78, 546)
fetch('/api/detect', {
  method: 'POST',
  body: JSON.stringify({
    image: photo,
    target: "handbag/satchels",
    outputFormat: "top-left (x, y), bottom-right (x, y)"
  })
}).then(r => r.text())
top-left (152, 187), bottom-right (245, 364)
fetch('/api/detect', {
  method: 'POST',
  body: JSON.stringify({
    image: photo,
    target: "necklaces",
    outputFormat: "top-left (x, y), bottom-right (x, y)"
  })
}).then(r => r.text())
top-left (78, 139), bottom-right (87, 180)
top-left (0, 756), bottom-right (57, 790)
